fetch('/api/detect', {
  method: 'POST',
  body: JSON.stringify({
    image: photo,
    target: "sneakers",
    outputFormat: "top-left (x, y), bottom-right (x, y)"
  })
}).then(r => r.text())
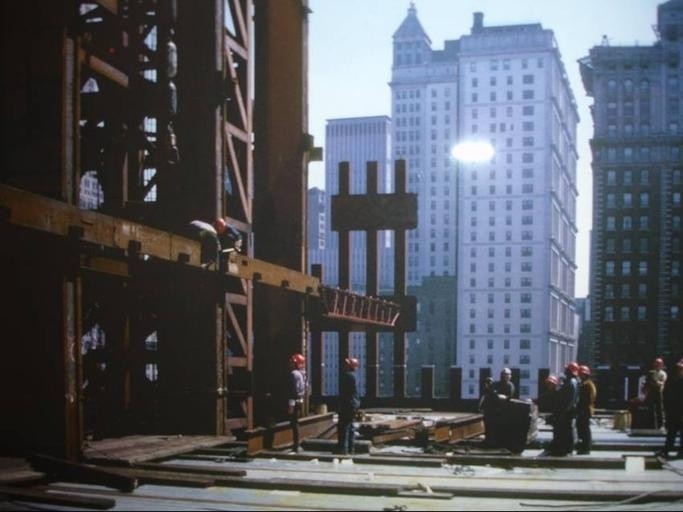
top-left (333, 448), bottom-right (355, 455)
top-left (288, 450), bottom-right (297, 455)
top-left (574, 441), bottom-right (590, 453)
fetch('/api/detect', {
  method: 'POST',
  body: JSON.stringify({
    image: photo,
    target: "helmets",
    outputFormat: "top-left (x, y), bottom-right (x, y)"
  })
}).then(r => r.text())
top-left (215, 218), bottom-right (225, 234)
top-left (290, 354), bottom-right (306, 368)
top-left (545, 376), bottom-right (560, 386)
top-left (654, 359), bottom-right (663, 366)
top-left (502, 368), bottom-right (512, 376)
top-left (568, 362), bottom-right (579, 376)
top-left (579, 366), bottom-right (591, 375)
top-left (345, 358), bottom-right (359, 368)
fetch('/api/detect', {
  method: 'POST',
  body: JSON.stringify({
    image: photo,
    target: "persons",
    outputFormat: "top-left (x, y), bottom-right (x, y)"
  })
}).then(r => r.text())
top-left (331, 356), bottom-right (361, 455)
top-left (284, 352), bottom-right (308, 416)
top-left (542, 361), bottom-right (596, 456)
top-left (657, 357), bottom-right (683, 461)
top-left (214, 213), bottom-right (246, 254)
top-left (487, 367), bottom-right (516, 399)
top-left (188, 220), bottom-right (221, 252)
top-left (642, 356), bottom-right (669, 430)
